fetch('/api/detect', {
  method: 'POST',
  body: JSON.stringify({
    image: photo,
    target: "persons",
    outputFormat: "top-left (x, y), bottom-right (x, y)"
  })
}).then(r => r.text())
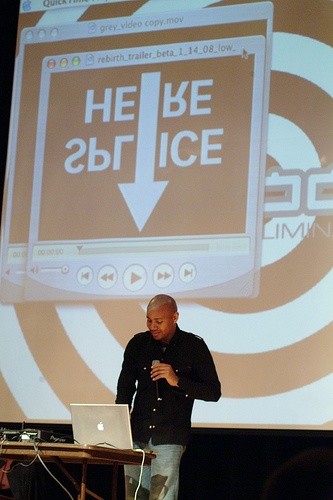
top-left (115, 294), bottom-right (221, 500)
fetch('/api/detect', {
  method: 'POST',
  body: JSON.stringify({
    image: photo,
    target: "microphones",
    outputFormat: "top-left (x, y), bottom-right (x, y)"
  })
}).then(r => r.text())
top-left (152, 360), bottom-right (163, 409)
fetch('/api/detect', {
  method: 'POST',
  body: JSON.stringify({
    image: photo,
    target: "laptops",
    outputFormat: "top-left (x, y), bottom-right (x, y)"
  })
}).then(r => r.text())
top-left (70, 404), bottom-right (134, 451)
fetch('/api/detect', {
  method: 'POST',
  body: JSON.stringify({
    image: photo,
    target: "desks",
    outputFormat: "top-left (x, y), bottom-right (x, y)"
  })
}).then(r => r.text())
top-left (0, 441), bottom-right (156, 500)
